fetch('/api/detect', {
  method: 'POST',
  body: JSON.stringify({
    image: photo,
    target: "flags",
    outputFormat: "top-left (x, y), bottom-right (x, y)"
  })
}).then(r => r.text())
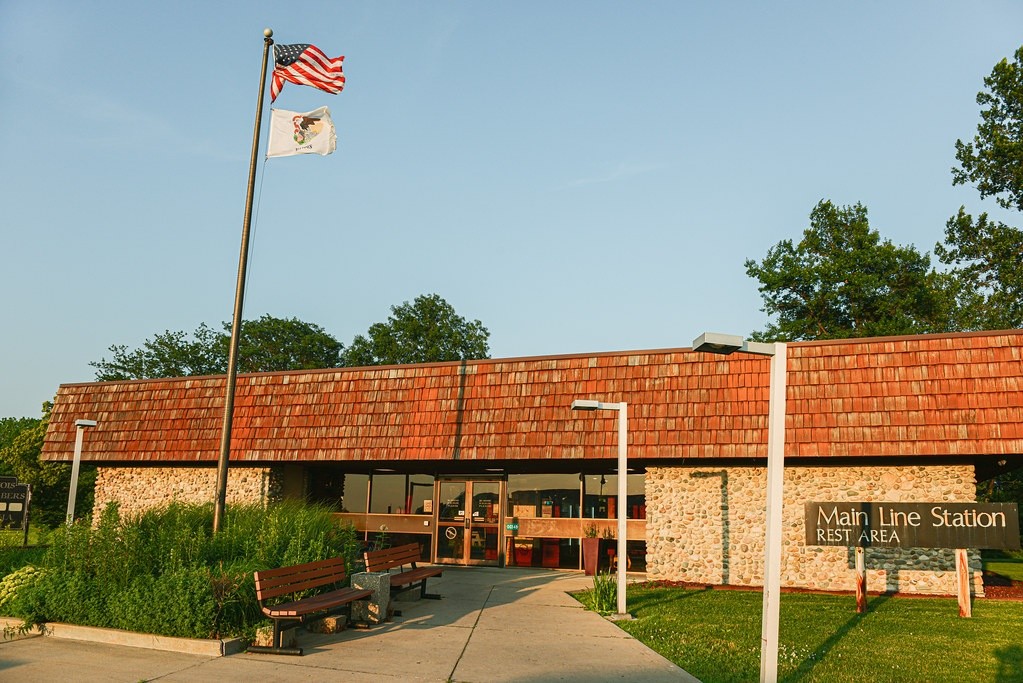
top-left (271, 43), bottom-right (345, 104)
top-left (266, 105), bottom-right (336, 159)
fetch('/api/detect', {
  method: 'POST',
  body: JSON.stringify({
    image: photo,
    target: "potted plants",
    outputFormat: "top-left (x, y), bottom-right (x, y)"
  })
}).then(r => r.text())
top-left (583, 523), bottom-right (603, 576)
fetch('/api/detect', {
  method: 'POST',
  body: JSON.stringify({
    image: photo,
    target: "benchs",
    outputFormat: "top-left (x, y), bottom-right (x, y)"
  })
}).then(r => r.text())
top-left (247, 557), bottom-right (375, 656)
top-left (364, 542), bottom-right (444, 617)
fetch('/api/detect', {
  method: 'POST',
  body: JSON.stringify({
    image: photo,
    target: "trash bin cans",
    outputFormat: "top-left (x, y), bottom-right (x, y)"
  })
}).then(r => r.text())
top-left (348, 571), bottom-right (390, 624)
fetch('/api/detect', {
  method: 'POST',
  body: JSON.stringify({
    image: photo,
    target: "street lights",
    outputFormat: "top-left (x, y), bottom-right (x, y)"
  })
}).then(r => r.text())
top-left (571, 398), bottom-right (629, 615)
top-left (691, 331), bottom-right (787, 683)
top-left (65, 419), bottom-right (98, 531)
